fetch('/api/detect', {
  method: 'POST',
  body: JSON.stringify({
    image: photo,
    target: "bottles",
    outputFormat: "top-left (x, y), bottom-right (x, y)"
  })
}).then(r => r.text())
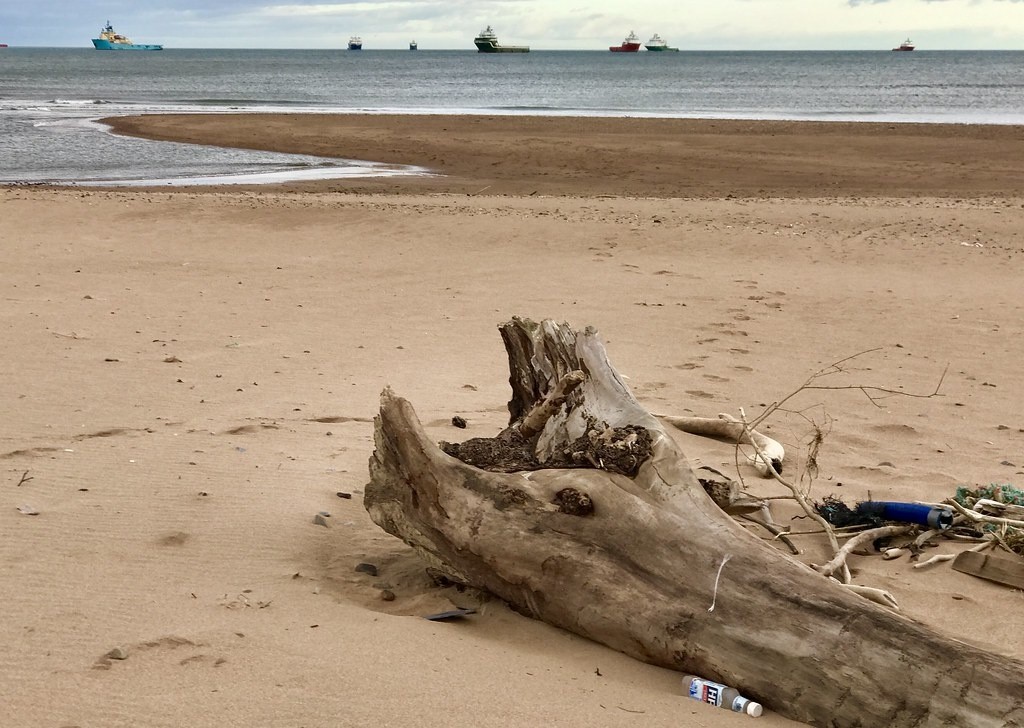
top-left (681, 674), bottom-right (763, 717)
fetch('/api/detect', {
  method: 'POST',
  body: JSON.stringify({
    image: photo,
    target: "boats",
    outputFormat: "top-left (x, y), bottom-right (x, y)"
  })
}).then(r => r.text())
top-left (347, 35), bottom-right (362, 50)
top-left (608, 30), bottom-right (642, 53)
top-left (891, 37), bottom-right (915, 51)
top-left (474, 25), bottom-right (531, 53)
top-left (91, 19), bottom-right (165, 51)
top-left (409, 40), bottom-right (418, 51)
top-left (644, 33), bottom-right (680, 52)
